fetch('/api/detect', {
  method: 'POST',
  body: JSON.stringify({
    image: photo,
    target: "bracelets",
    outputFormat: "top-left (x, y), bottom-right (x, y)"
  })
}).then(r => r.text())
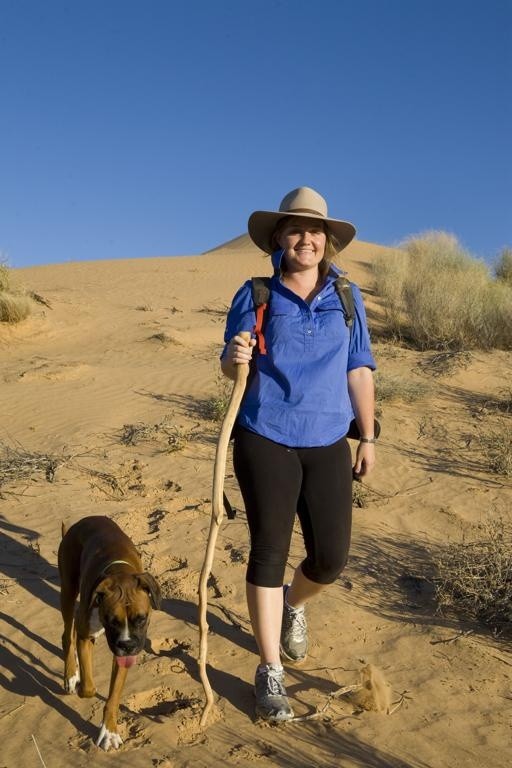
top-left (359, 437), bottom-right (374, 445)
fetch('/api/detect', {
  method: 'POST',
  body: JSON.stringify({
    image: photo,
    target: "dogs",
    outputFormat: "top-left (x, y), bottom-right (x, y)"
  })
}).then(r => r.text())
top-left (57, 515), bottom-right (163, 753)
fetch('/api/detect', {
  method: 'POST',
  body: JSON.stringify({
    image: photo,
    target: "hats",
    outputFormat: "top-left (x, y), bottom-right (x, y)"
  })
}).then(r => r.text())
top-left (248, 186), bottom-right (356, 256)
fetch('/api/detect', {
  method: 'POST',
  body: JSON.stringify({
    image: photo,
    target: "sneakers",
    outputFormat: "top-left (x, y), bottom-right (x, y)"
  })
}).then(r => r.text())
top-left (254, 583), bottom-right (308, 720)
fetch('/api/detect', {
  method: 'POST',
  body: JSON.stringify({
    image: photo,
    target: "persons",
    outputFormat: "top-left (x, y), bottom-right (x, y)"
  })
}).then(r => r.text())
top-left (220, 186), bottom-right (376, 720)
